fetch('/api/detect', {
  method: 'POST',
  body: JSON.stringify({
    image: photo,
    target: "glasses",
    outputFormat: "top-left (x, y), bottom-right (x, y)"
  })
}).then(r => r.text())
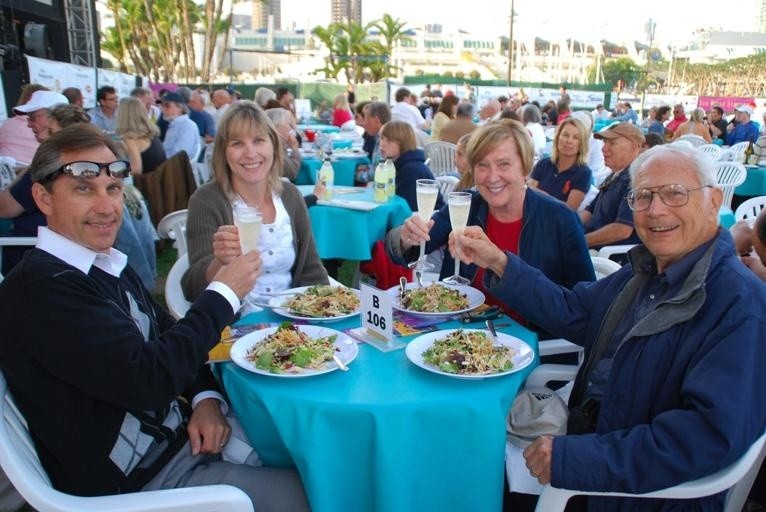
top-left (36, 160), bottom-right (132, 186)
top-left (624, 182), bottom-right (715, 214)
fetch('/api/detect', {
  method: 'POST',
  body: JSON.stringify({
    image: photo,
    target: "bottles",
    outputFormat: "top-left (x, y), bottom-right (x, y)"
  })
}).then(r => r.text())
top-left (375, 158), bottom-right (389, 203)
top-left (320, 158), bottom-right (333, 201)
top-left (384, 157), bottom-right (396, 198)
top-left (354, 161), bottom-right (370, 188)
top-left (745, 135), bottom-right (754, 165)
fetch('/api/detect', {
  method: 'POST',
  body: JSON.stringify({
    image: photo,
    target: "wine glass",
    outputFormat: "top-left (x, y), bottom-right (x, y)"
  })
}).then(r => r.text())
top-left (406, 178), bottom-right (440, 272)
top-left (442, 191), bottom-right (473, 286)
top-left (232, 203), bottom-right (257, 303)
top-left (237, 213), bottom-right (268, 313)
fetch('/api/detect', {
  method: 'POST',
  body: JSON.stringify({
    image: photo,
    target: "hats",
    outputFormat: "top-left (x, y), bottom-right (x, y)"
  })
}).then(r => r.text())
top-left (154, 92), bottom-right (186, 106)
top-left (593, 121), bottom-right (648, 147)
top-left (504, 384), bottom-right (570, 451)
top-left (733, 105), bottom-right (754, 117)
top-left (10, 89), bottom-right (71, 118)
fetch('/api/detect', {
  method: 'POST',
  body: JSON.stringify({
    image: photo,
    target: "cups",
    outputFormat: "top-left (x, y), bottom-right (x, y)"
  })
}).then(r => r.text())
top-left (748, 153), bottom-right (758, 165)
top-left (736, 153), bottom-right (746, 165)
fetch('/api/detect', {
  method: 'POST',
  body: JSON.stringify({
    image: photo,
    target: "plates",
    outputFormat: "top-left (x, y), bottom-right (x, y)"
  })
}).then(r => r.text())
top-left (232, 325), bottom-right (359, 376)
top-left (387, 279), bottom-right (485, 321)
top-left (271, 287), bottom-right (366, 320)
top-left (401, 327), bottom-right (536, 383)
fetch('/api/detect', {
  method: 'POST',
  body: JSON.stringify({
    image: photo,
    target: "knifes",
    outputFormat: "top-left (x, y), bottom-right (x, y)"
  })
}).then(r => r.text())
top-left (414, 301), bottom-right (500, 330)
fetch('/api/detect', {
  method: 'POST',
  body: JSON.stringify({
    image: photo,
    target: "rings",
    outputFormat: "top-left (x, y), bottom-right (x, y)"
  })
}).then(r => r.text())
top-left (219, 441), bottom-right (226, 449)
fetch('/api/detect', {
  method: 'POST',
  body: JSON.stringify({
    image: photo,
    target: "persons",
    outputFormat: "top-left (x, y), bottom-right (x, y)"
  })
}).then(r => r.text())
top-left (383, 117), bottom-right (600, 394)
top-left (448, 136), bottom-right (766, 512)
top-left (1, 121), bottom-right (312, 511)
top-left (1, 73), bottom-right (241, 274)
top-left (254, 86), bottom-right (546, 289)
top-left (525, 85), bottom-right (766, 280)
